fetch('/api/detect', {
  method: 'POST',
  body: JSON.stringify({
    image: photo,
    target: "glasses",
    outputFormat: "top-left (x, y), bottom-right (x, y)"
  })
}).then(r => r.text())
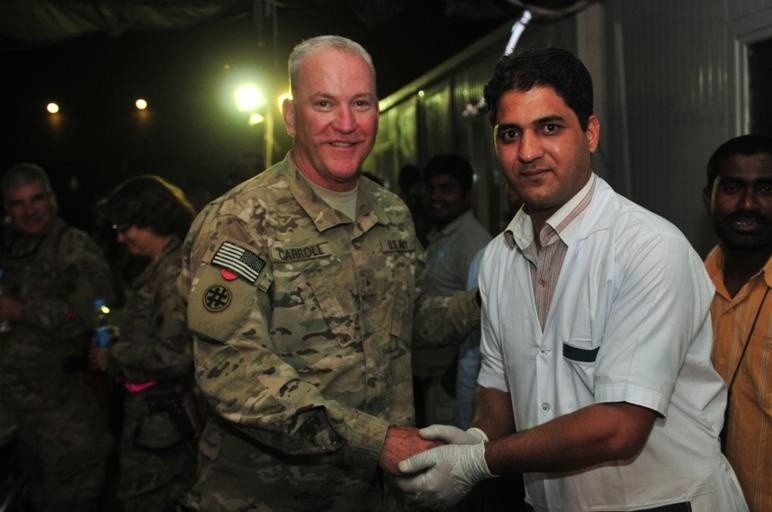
top-left (112, 223), bottom-right (137, 233)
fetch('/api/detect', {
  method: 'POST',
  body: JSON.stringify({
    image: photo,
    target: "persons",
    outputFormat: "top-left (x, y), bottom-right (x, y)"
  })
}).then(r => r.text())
top-left (360, 153), bottom-right (534, 512)
top-left (0, 161), bottom-right (123, 510)
top-left (85, 171), bottom-right (207, 511)
top-left (384, 46), bottom-right (753, 512)
top-left (700, 131), bottom-right (771, 511)
top-left (172, 35), bottom-right (485, 510)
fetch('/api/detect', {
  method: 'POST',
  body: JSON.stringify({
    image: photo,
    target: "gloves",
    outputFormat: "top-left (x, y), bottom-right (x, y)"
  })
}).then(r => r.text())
top-left (392, 422), bottom-right (500, 510)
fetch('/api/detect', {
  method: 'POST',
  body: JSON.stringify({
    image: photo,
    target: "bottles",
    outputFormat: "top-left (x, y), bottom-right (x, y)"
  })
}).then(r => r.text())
top-left (94, 300), bottom-right (114, 345)
